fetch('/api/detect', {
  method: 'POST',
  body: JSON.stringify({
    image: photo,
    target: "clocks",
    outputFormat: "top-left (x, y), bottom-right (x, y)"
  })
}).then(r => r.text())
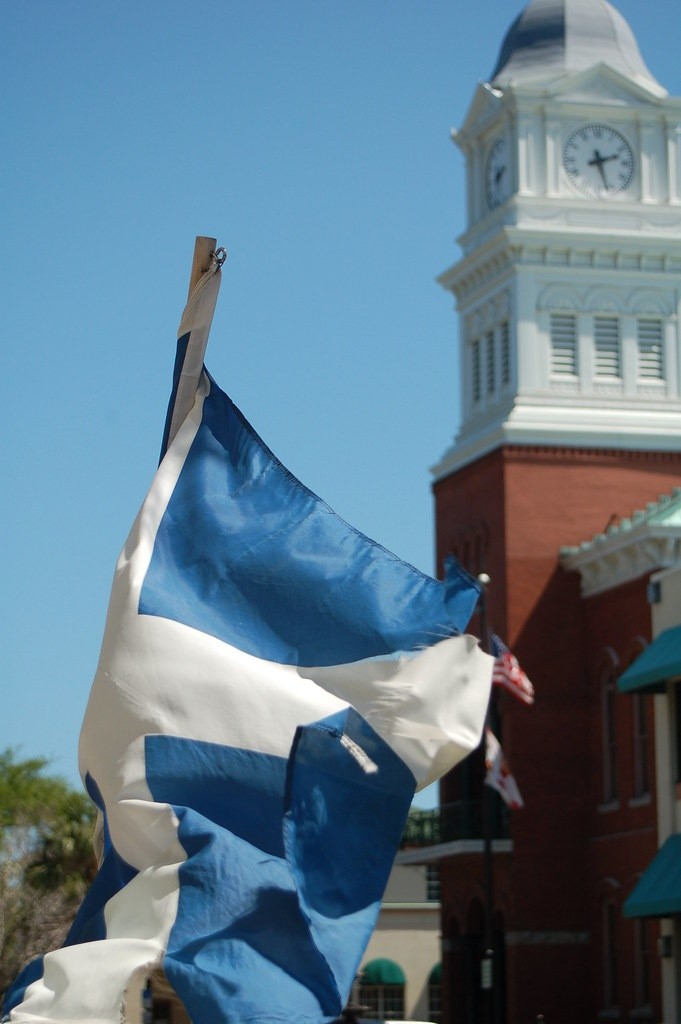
top-left (484, 141), bottom-right (514, 208)
top-left (562, 126), bottom-right (632, 198)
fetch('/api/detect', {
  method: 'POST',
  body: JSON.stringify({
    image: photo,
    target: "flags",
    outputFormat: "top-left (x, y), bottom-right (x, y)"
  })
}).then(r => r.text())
top-left (0, 264), bottom-right (533, 1023)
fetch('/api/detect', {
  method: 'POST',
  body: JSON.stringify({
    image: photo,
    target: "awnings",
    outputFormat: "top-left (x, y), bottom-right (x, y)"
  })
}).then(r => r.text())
top-left (622, 832), bottom-right (681, 921)
top-left (614, 624), bottom-right (681, 695)
